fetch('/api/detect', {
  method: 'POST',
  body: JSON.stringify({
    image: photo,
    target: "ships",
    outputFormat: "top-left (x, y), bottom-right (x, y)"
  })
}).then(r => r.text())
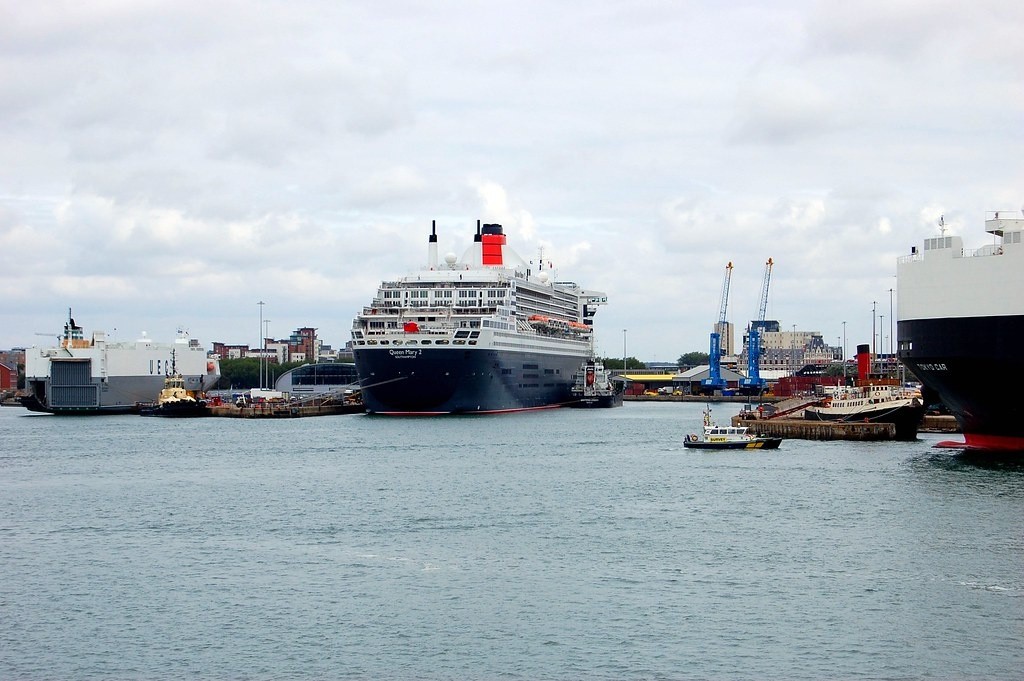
top-left (350, 220), bottom-right (608, 417)
top-left (897, 211), bottom-right (1024, 449)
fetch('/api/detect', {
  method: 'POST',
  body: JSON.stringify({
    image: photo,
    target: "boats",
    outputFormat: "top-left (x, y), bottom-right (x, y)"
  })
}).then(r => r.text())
top-left (137, 348), bottom-right (364, 418)
top-left (683, 401), bottom-right (783, 450)
top-left (275, 364), bottom-right (362, 398)
top-left (570, 359), bottom-right (628, 409)
top-left (14, 307), bottom-right (220, 416)
top-left (804, 382), bottom-right (925, 441)
top-left (731, 405), bottom-right (895, 439)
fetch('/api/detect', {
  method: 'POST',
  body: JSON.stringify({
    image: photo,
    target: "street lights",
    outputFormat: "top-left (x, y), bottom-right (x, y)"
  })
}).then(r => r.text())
top-left (886, 289), bottom-right (896, 379)
top-left (622, 329), bottom-right (627, 394)
top-left (870, 301), bottom-right (878, 370)
top-left (791, 324), bottom-right (798, 377)
top-left (257, 300), bottom-right (269, 391)
top-left (879, 315), bottom-right (884, 379)
top-left (842, 322), bottom-right (847, 377)
